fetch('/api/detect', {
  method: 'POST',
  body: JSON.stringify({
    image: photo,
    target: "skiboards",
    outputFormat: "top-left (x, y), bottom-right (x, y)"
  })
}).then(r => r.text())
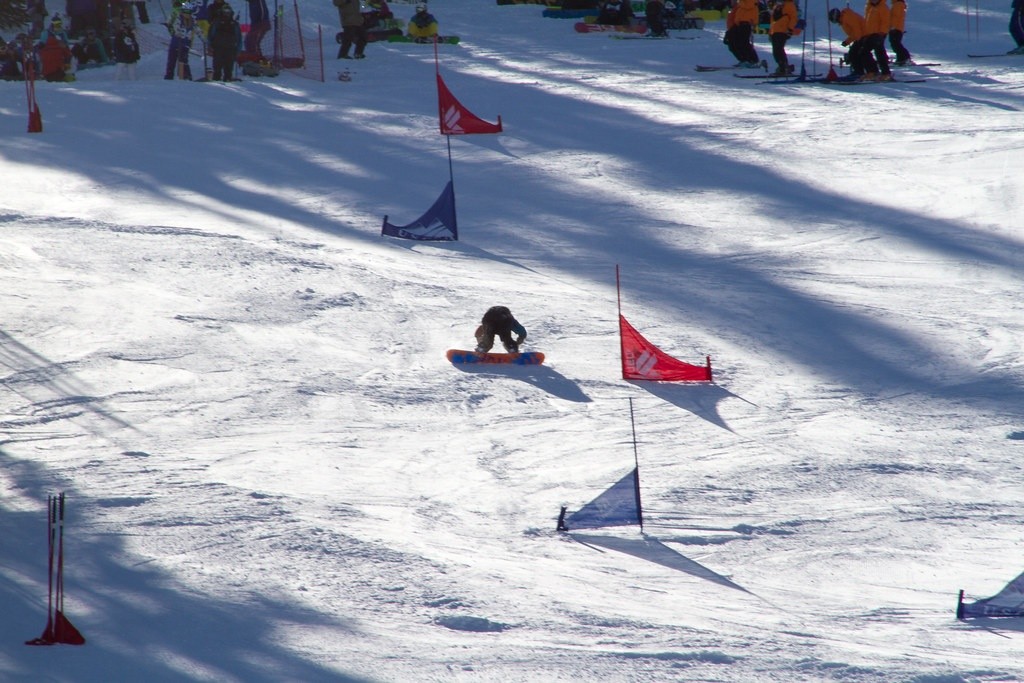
top-left (968, 54), bottom-right (1008, 58)
top-left (694, 63), bottom-right (736, 72)
top-left (762, 79), bottom-right (857, 84)
top-left (733, 73), bottom-right (823, 78)
top-left (910, 63), bottom-right (941, 67)
top-left (820, 79), bottom-right (926, 85)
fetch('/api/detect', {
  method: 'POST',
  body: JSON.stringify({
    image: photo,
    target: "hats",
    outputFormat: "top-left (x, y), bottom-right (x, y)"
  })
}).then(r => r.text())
top-left (50, 15), bottom-right (63, 25)
top-left (221, 3), bottom-right (232, 12)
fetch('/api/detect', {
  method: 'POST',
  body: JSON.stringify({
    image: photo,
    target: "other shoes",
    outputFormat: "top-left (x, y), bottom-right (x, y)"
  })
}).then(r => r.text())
top-left (895, 51), bottom-right (910, 66)
top-left (504, 340), bottom-right (518, 353)
top-left (337, 55), bottom-right (352, 59)
top-left (846, 72), bottom-right (865, 80)
top-left (183, 74), bottom-right (192, 81)
top-left (354, 54), bottom-right (365, 59)
top-left (877, 73), bottom-right (892, 82)
top-left (776, 64), bottom-right (795, 74)
top-left (164, 73), bottom-right (175, 80)
top-left (474, 341), bottom-right (489, 353)
top-left (860, 72), bottom-right (880, 81)
top-left (732, 54), bottom-right (759, 67)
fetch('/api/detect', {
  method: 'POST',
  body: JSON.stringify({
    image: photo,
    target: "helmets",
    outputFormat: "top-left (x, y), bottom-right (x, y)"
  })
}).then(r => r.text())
top-left (416, 2), bottom-right (428, 11)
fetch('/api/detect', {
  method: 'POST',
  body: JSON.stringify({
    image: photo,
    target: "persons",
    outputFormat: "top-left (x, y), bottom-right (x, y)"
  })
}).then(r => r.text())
top-left (474, 305), bottom-right (527, 354)
top-left (1006, 0), bottom-right (1024, 56)
top-left (858, 0), bottom-right (894, 81)
top-left (0, 0), bottom-right (809, 84)
top-left (888, 0), bottom-right (912, 67)
top-left (828, 7), bottom-right (881, 78)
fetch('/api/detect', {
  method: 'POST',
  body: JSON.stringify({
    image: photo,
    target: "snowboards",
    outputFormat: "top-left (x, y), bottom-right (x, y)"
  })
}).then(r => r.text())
top-left (630, 1), bottom-right (733, 21)
top-left (45, 72), bottom-right (75, 82)
top-left (574, 14), bottom-right (705, 34)
top-left (751, 9), bottom-right (807, 35)
top-left (388, 33), bottom-right (460, 44)
top-left (541, 9), bottom-right (600, 19)
top-left (335, 28), bottom-right (402, 44)
top-left (76, 63), bottom-right (115, 70)
top-left (445, 348), bottom-right (546, 365)
top-left (609, 35), bottom-right (701, 40)
